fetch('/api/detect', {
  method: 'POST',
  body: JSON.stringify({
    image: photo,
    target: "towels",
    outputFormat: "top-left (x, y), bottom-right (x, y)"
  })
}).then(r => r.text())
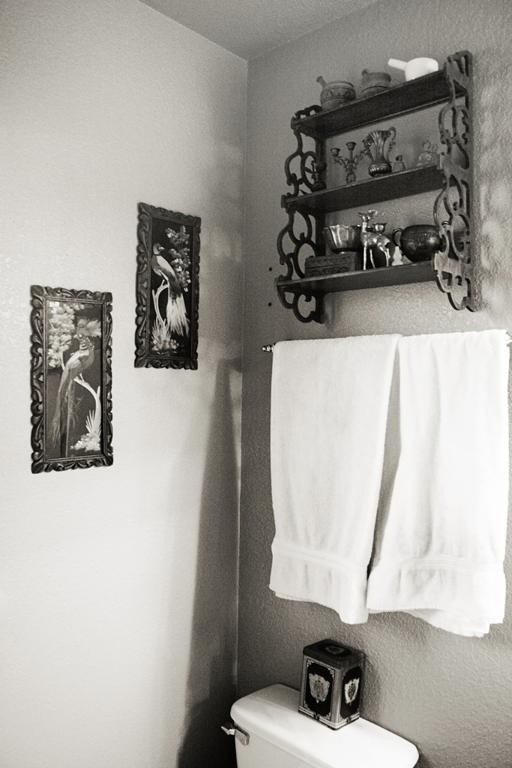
top-left (266, 330), bottom-right (403, 626)
top-left (363, 330), bottom-right (511, 639)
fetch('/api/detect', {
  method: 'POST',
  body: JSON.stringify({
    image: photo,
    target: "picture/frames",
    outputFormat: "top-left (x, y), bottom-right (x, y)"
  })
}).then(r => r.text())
top-left (136, 204), bottom-right (202, 370)
top-left (30, 285), bottom-right (113, 472)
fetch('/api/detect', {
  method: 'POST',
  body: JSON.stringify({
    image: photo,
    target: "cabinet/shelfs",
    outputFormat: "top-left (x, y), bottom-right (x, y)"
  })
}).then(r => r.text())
top-left (275, 50), bottom-right (477, 325)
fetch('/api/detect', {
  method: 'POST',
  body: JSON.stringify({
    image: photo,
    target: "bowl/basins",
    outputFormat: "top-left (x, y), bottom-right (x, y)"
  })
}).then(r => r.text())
top-left (322, 224), bottom-right (362, 253)
